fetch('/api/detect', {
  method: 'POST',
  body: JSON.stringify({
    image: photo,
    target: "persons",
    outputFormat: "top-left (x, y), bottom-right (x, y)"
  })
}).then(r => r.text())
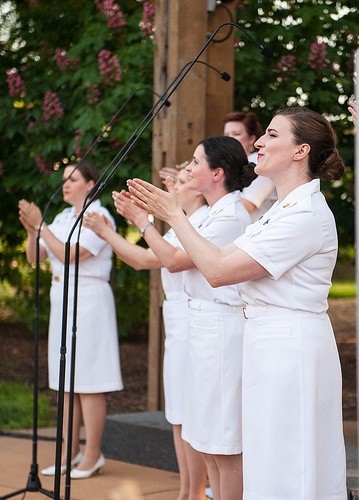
top-left (82, 167), bottom-right (210, 500)
top-left (158, 111), bottom-right (279, 222)
top-left (126, 106), bottom-right (348, 500)
top-left (112, 136), bottom-right (259, 500)
top-left (18, 162), bottom-right (115, 478)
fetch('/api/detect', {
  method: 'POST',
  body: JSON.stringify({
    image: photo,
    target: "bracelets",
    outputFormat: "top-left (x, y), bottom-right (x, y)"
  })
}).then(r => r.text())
top-left (35, 223), bottom-right (47, 233)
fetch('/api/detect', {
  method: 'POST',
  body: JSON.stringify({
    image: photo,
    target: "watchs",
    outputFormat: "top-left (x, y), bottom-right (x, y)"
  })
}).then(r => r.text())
top-left (139, 222), bottom-right (153, 237)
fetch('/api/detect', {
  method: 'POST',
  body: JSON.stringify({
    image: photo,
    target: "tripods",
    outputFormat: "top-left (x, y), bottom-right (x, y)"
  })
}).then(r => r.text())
top-left (0, 240), bottom-right (55, 500)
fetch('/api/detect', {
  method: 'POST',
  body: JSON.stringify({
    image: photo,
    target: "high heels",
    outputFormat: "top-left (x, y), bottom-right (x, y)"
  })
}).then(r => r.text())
top-left (41, 450), bottom-right (84, 476)
top-left (64, 452), bottom-right (105, 478)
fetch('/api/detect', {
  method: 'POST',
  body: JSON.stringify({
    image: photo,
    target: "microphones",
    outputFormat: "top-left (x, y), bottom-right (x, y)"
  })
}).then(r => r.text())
top-left (38, 21), bottom-right (273, 242)
